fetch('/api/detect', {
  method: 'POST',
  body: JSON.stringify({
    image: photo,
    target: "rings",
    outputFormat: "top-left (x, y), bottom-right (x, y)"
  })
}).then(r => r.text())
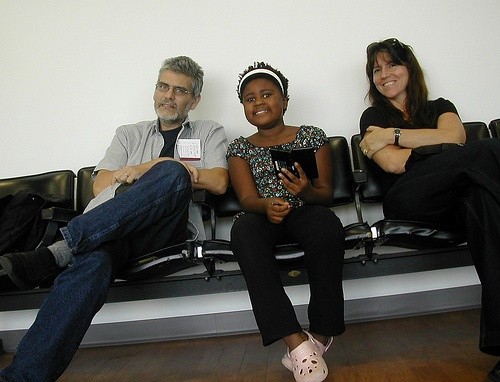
top-left (363, 150), bottom-right (367, 155)
top-left (124, 173), bottom-right (129, 177)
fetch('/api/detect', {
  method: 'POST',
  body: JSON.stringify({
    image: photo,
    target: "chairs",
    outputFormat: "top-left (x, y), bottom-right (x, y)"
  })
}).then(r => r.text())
top-left (0, 121), bottom-right (500, 296)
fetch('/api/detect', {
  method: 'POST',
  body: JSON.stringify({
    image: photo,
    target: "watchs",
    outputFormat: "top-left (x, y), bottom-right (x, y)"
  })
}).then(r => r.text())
top-left (392, 128), bottom-right (403, 146)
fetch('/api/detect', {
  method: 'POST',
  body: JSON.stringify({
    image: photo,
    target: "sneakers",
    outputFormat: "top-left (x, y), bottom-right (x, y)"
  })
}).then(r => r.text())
top-left (0, 248), bottom-right (62, 293)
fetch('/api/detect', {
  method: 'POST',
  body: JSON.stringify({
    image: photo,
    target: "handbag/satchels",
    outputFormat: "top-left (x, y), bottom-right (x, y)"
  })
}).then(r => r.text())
top-left (0, 190), bottom-right (71, 255)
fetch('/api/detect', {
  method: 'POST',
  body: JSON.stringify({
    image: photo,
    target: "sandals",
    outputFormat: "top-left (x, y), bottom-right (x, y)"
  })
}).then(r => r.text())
top-left (282, 336), bottom-right (333, 372)
top-left (286, 330), bottom-right (329, 382)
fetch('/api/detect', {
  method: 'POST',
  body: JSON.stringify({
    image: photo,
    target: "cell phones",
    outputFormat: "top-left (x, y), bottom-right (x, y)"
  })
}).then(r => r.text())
top-left (271, 150), bottom-right (291, 180)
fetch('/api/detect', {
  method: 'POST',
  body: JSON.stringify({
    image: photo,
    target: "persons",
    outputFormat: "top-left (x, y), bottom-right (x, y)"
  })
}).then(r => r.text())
top-left (227, 61), bottom-right (347, 382)
top-left (359, 38), bottom-right (500, 382)
top-left (0, 56), bottom-right (230, 382)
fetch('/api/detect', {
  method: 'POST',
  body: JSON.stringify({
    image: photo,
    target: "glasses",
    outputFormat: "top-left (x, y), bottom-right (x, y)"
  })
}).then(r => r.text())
top-left (366, 37), bottom-right (404, 58)
top-left (155, 83), bottom-right (198, 96)
